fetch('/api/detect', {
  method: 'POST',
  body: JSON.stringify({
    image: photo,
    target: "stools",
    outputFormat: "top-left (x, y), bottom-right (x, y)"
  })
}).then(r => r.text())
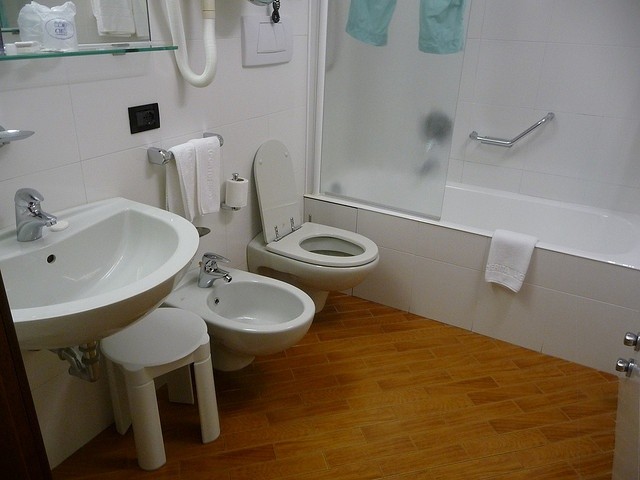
top-left (99, 307), bottom-right (221, 472)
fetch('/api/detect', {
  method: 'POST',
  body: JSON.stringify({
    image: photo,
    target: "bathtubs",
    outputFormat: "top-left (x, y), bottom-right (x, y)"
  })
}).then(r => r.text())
top-left (438, 182), bottom-right (640, 271)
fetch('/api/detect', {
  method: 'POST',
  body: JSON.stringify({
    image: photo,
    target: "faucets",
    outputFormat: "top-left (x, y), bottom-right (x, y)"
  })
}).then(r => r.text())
top-left (14, 187), bottom-right (57, 241)
top-left (197, 252), bottom-right (232, 290)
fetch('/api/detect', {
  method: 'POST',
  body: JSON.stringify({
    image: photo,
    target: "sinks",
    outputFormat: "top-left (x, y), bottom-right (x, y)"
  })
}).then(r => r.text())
top-left (164, 268), bottom-right (315, 372)
top-left (1, 197), bottom-right (199, 350)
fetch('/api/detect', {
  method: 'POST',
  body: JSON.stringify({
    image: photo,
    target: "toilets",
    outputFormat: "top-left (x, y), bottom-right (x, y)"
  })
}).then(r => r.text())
top-left (247, 140), bottom-right (379, 314)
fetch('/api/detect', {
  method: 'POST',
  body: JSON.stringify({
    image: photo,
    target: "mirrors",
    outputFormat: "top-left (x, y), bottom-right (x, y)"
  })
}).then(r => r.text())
top-left (0, 1), bottom-right (152, 50)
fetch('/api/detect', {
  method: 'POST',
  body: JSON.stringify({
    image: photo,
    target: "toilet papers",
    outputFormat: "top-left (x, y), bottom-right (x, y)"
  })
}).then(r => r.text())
top-left (225, 177), bottom-right (249, 208)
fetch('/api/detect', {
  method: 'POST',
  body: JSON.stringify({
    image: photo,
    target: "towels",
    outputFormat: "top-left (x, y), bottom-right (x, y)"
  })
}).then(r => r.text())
top-left (92, 1), bottom-right (135, 39)
top-left (417, 0), bottom-right (465, 53)
top-left (484, 229), bottom-right (541, 293)
top-left (166, 141), bottom-right (198, 221)
top-left (188, 137), bottom-right (221, 215)
top-left (345, 0), bottom-right (398, 47)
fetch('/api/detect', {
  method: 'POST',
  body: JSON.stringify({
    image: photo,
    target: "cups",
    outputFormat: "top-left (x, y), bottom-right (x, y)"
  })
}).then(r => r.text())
top-left (3, 44), bottom-right (17, 57)
